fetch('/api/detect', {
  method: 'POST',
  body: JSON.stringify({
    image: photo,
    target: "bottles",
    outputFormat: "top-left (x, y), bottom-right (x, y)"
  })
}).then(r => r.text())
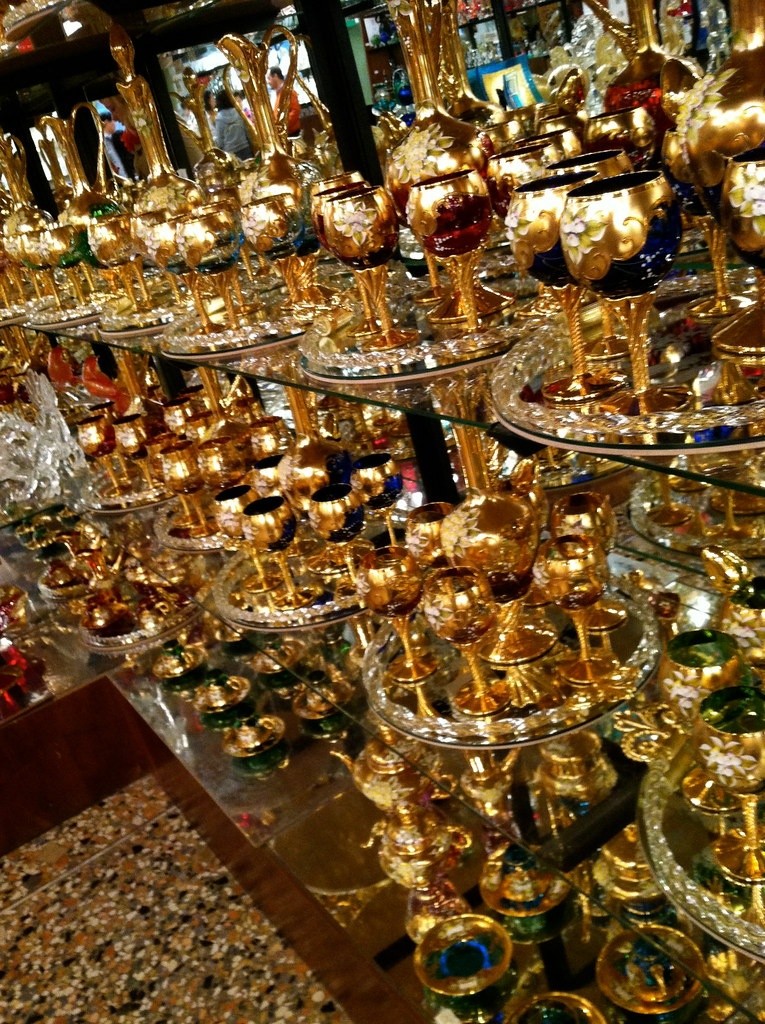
top-left (192, 366), bottom-right (254, 480)
top-left (439, 421), bottom-right (542, 602)
top-left (39, 102), bottom-right (126, 269)
top-left (272, 384), bottom-right (357, 543)
top-left (114, 78), bottom-right (208, 275)
top-left (108, 347), bottom-right (168, 448)
top-left (365, 0), bottom-right (765, 354)
top-left (216, 23), bottom-right (327, 311)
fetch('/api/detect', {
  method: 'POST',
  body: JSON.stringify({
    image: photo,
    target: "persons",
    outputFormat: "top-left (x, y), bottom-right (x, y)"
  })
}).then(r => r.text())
top-left (266, 66), bottom-right (301, 137)
top-left (187, 91), bottom-right (253, 160)
top-left (100, 112), bottom-right (134, 181)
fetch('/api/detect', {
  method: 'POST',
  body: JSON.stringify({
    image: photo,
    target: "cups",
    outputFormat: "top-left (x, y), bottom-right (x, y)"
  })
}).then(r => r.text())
top-left (708, 149), bottom-right (765, 274)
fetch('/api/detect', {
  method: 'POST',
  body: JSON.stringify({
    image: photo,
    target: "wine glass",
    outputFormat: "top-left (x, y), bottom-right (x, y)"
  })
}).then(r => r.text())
top-left (3, 192), bottom-right (312, 336)
top-left (695, 685), bottom-right (765, 883)
top-left (78, 384), bottom-right (409, 612)
top-left (659, 627), bottom-right (756, 812)
top-left (317, 167), bottom-right (500, 354)
top-left (361, 492), bottom-right (631, 720)
top-left (492, 106), bottom-right (693, 419)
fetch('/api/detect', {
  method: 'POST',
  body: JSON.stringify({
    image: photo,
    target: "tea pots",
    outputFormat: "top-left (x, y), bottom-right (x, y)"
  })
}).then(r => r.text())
top-left (329, 722), bottom-right (492, 947)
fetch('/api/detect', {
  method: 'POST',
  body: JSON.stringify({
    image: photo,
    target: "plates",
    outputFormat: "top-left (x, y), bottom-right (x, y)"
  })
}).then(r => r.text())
top-left (637, 728), bottom-right (765, 965)
top-left (0, 298), bottom-right (28, 327)
top-left (98, 299), bottom-right (176, 339)
top-left (79, 467), bottom-right (177, 515)
top-left (154, 498), bottom-right (232, 553)
top-left (217, 549), bottom-right (363, 635)
top-left (159, 290), bottom-right (306, 360)
top-left (300, 277), bottom-right (543, 384)
top-left (28, 296), bottom-right (99, 331)
top-left (359, 591), bottom-right (660, 748)
top-left (491, 268), bottom-right (765, 457)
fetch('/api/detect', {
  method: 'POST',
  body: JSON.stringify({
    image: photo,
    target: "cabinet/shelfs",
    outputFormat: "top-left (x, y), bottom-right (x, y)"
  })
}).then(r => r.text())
top-left (0, 0), bottom-right (765, 1024)
